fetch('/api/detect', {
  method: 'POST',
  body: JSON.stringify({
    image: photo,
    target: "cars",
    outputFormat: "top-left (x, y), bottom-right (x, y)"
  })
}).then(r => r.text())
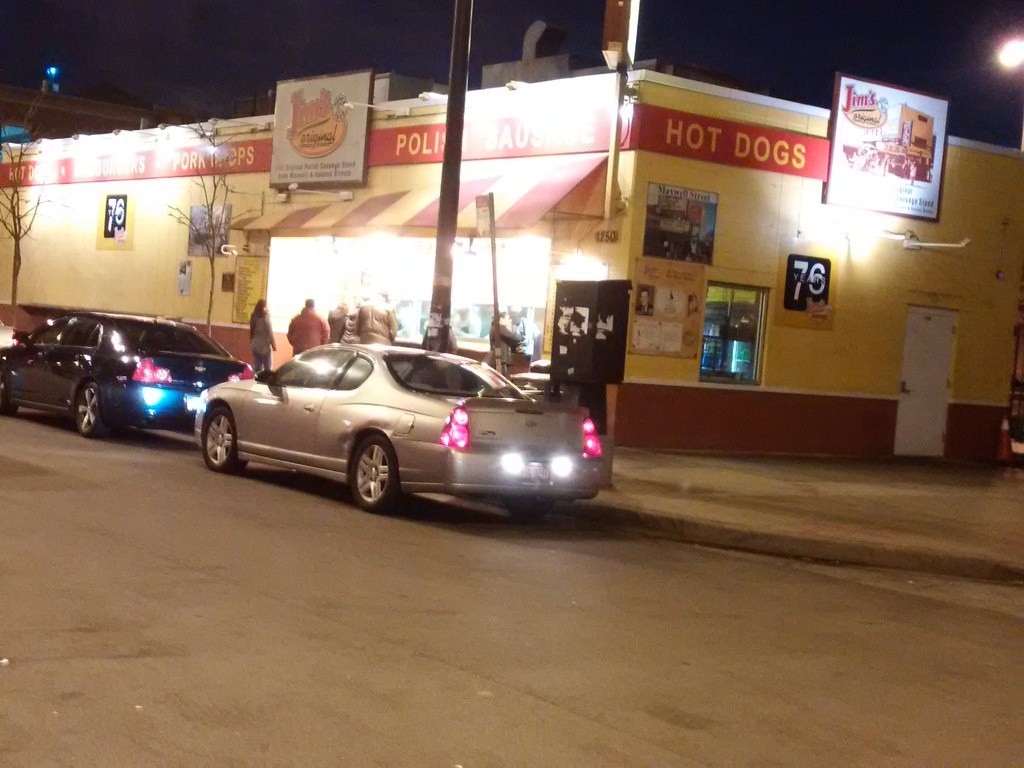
top-left (193, 340), bottom-right (607, 520)
top-left (0, 311), bottom-right (255, 442)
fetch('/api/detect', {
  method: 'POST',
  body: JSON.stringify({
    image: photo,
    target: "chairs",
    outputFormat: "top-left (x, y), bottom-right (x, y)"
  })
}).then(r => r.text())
top-left (131, 329), bottom-right (172, 349)
top-left (62, 327), bottom-right (83, 345)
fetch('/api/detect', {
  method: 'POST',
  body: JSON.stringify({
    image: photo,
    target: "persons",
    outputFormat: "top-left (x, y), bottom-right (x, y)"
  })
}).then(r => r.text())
top-left (635, 289), bottom-right (653, 315)
top-left (250, 284), bottom-right (541, 373)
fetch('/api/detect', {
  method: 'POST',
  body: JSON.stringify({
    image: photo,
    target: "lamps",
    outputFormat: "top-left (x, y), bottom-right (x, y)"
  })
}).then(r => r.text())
top-left (208, 117), bottom-right (271, 131)
top-left (342, 101), bottom-right (393, 113)
top-left (113, 128), bottom-right (170, 140)
top-left (221, 244), bottom-right (258, 258)
top-left (1, 142), bottom-right (9, 148)
top-left (507, 79), bottom-right (530, 94)
top-left (72, 134), bottom-right (113, 141)
top-left (276, 193), bottom-right (288, 202)
top-left (418, 90), bottom-right (449, 104)
top-left (288, 182), bottom-right (355, 200)
top-left (35, 136), bottom-right (43, 144)
top-left (157, 122), bottom-right (217, 135)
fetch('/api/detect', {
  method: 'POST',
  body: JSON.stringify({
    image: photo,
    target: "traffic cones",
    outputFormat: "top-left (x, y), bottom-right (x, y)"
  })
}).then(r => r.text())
top-left (997, 415), bottom-right (1018, 464)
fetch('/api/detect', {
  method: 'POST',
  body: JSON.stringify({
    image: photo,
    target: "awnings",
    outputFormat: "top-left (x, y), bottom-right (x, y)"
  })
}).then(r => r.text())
top-left (227, 151), bottom-right (607, 238)
